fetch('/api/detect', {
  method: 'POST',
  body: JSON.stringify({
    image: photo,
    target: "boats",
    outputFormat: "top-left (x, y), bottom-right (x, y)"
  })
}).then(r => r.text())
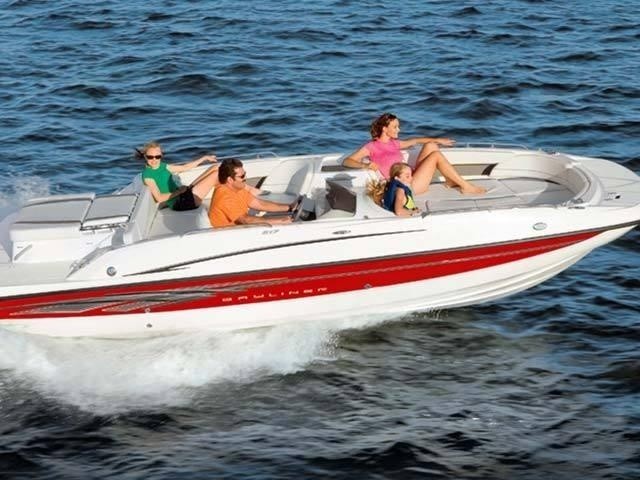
top-left (0, 140), bottom-right (640, 340)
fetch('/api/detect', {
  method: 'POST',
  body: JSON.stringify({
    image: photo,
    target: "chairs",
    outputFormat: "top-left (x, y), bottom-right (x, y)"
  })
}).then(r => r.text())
top-left (194, 205), bottom-right (213, 230)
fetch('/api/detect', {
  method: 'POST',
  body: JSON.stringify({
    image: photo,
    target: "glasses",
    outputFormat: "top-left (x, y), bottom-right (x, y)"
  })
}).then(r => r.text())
top-left (234, 172), bottom-right (247, 178)
top-left (146, 155), bottom-right (162, 159)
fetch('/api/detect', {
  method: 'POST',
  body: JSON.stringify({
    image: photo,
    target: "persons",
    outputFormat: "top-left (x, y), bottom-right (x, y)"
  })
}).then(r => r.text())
top-left (208, 157), bottom-right (301, 227)
top-left (141, 141), bottom-right (272, 211)
top-left (342, 113), bottom-right (486, 196)
top-left (383, 161), bottom-right (423, 217)
